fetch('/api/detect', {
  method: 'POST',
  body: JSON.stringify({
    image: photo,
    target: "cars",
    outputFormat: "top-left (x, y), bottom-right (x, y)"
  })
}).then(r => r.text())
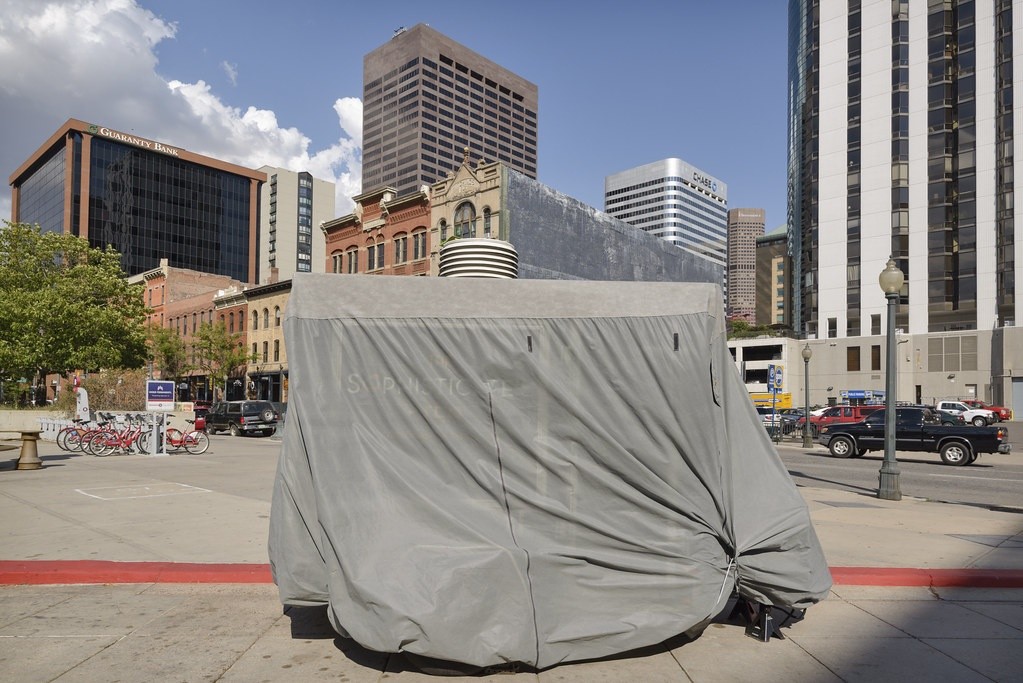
top-left (191, 400), bottom-right (213, 418)
top-left (777, 408), bottom-right (803, 432)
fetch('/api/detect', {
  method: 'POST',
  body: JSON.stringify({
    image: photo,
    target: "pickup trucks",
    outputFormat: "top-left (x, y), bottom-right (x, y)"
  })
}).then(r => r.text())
top-left (799, 407), bottom-right (885, 438)
top-left (963, 400), bottom-right (1013, 423)
top-left (936, 401), bottom-right (997, 427)
top-left (818, 406), bottom-right (1012, 466)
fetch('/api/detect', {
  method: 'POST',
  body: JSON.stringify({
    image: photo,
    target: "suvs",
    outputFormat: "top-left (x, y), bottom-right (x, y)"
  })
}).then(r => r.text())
top-left (205, 400), bottom-right (279, 436)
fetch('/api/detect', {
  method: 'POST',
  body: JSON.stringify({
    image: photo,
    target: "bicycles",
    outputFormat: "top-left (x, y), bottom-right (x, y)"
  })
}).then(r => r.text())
top-left (57, 414), bottom-right (210, 457)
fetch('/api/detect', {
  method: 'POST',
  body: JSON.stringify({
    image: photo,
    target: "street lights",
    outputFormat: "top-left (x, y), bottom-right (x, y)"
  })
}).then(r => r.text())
top-left (223, 373), bottom-right (228, 400)
top-left (876, 254), bottom-right (905, 500)
top-left (802, 343), bottom-right (813, 448)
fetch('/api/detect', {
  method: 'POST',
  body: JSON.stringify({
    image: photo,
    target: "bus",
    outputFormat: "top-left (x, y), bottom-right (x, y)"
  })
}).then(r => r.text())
top-left (750, 392), bottom-right (792, 408)
top-left (755, 404), bottom-right (782, 434)
